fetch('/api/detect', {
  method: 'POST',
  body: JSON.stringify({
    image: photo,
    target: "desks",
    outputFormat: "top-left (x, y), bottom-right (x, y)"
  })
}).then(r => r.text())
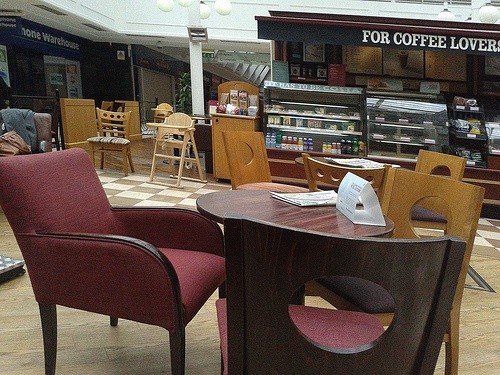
top-left (295, 157), bottom-right (402, 181)
top-left (196, 188), bottom-right (396, 238)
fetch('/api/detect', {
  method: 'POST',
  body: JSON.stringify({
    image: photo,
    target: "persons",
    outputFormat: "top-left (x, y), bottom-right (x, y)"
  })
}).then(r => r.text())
top-left (0, 76), bottom-right (9, 109)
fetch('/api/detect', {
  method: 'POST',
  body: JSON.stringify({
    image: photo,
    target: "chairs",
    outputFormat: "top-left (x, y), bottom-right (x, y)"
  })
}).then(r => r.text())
top-left (87, 107), bottom-right (135, 177)
top-left (0, 147), bottom-right (227, 375)
top-left (215, 149), bottom-right (484, 375)
top-left (0, 112), bottom-right (51, 154)
top-left (32, 98), bottom-right (60, 151)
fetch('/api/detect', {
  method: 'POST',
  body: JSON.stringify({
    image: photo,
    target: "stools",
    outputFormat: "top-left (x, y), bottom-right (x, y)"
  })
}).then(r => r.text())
top-left (152, 103), bottom-right (173, 142)
top-left (145, 112), bottom-right (207, 188)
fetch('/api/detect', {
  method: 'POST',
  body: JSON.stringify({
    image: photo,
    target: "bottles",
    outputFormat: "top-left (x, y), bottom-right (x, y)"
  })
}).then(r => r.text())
top-left (322, 137), bottom-right (365, 157)
top-left (266, 132), bottom-right (313, 152)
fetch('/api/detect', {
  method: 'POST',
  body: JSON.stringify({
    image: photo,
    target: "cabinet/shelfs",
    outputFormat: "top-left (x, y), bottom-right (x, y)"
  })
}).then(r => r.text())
top-left (449, 100), bottom-right (489, 169)
top-left (260, 80), bottom-right (364, 189)
top-left (211, 112), bottom-right (262, 182)
top-left (363, 87), bottom-right (449, 158)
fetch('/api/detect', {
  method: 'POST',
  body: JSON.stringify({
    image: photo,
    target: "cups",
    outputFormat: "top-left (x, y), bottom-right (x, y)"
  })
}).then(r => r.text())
top-left (398, 50), bottom-right (408, 68)
